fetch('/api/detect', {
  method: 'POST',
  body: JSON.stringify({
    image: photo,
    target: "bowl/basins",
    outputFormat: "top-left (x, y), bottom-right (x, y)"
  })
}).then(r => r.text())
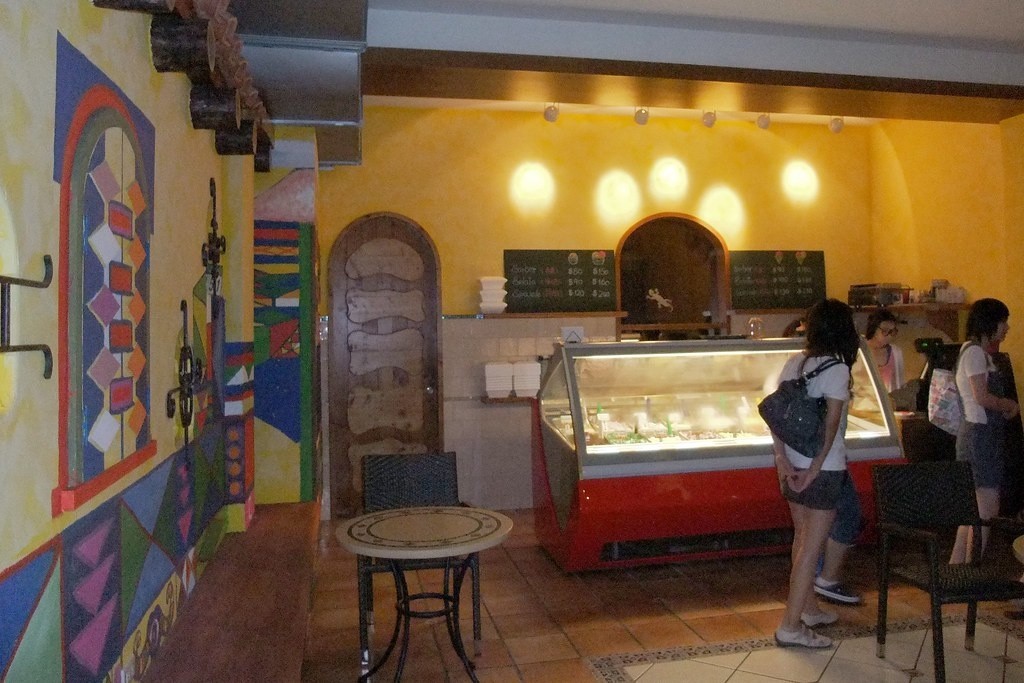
top-left (484, 360), bottom-right (542, 398)
top-left (477, 275), bottom-right (509, 315)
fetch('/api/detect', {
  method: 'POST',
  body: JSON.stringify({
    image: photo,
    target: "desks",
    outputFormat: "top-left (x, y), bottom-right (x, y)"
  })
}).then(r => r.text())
top-left (335, 504), bottom-right (513, 683)
top-left (140, 502), bottom-right (320, 683)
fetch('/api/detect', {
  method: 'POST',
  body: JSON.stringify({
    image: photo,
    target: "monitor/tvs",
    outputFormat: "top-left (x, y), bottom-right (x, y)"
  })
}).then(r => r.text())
top-left (921, 342), bottom-right (965, 380)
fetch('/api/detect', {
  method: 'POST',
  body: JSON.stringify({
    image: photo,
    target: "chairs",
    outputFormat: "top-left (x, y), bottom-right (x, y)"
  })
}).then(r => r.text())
top-left (871, 458), bottom-right (1023, 683)
top-left (358, 453), bottom-right (480, 657)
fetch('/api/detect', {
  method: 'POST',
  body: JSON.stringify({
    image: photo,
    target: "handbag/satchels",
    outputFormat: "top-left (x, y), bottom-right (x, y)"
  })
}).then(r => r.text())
top-left (759, 358), bottom-right (842, 458)
top-left (927, 341), bottom-right (989, 437)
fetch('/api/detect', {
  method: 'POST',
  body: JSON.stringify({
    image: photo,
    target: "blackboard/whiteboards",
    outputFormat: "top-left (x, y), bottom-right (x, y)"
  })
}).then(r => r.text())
top-left (731, 250), bottom-right (826, 310)
top-left (504, 250), bottom-right (617, 312)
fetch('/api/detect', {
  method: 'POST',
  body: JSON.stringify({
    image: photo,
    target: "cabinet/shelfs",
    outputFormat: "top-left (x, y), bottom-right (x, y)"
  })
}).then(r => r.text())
top-left (481, 312), bottom-right (625, 406)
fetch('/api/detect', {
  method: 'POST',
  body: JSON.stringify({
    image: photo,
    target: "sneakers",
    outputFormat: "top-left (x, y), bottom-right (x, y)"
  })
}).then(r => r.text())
top-left (813, 582), bottom-right (860, 602)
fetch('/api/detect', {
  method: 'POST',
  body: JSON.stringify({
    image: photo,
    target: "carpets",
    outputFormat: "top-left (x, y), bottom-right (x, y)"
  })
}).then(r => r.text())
top-left (582, 609), bottom-right (1024, 683)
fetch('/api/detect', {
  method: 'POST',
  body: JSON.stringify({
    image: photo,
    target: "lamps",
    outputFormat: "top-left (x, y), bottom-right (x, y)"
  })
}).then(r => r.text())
top-left (544, 102), bottom-right (560, 122)
top-left (757, 112), bottom-right (770, 129)
top-left (828, 116), bottom-right (845, 133)
top-left (702, 110), bottom-right (718, 128)
top-left (634, 105), bottom-right (649, 124)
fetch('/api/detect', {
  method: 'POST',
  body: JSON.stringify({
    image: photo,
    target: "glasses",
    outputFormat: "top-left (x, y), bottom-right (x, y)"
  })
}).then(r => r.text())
top-left (877, 325), bottom-right (899, 337)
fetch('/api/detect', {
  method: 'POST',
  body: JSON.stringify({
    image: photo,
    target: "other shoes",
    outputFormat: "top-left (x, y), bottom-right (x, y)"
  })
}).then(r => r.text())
top-left (774, 625), bottom-right (833, 648)
top-left (801, 609), bottom-right (839, 626)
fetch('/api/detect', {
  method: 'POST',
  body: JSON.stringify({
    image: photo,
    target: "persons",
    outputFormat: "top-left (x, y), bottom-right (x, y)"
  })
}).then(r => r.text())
top-left (782, 308), bottom-right (905, 413)
top-left (949, 298), bottom-right (1020, 566)
top-left (770, 297), bottom-right (861, 652)
